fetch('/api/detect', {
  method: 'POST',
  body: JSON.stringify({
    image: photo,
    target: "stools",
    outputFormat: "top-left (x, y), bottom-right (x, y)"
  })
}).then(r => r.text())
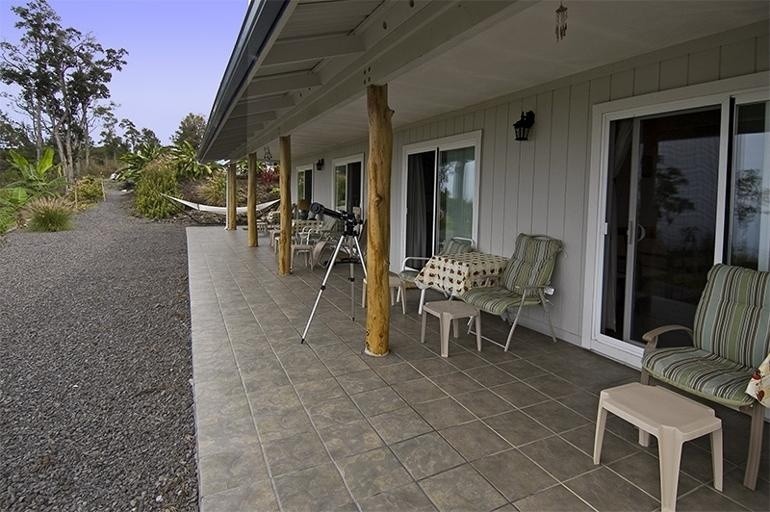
top-left (594, 381), bottom-right (724, 512)
top-left (362, 276), bottom-right (408, 316)
top-left (418, 299), bottom-right (483, 357)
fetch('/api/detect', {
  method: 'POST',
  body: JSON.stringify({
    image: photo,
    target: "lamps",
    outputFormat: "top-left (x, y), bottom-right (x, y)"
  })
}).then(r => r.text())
top-left (316, 159), bottom-right (324, 170)
top-left (513, 110), bottom-right (535, 141)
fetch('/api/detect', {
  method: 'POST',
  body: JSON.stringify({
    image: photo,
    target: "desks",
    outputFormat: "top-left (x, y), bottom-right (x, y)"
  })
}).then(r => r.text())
top-left (414, 252), bottom-right (511, 324)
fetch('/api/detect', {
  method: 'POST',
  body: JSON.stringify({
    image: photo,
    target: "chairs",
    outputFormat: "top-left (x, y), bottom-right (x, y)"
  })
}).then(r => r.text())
top-left (461, 233), bottom-right (563, 352)
top-left (394, 235), bottom-right (475, 314)
top-left (642, 264), bottom-right (770, 490)
top-left (255, 204), bottom-right (340, 273)
